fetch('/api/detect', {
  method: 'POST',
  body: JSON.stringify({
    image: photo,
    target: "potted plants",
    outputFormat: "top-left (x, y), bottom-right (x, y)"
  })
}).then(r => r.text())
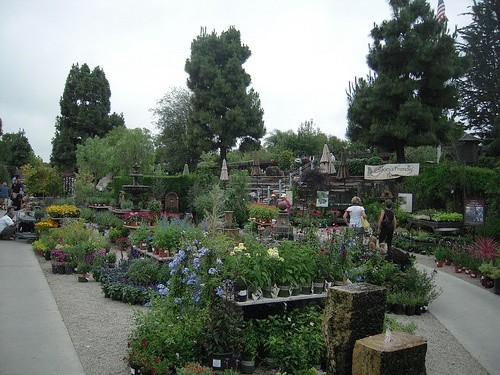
top-left (445, 248), bottom-right (494, 288)
top-left (385, 292), bottom-right (431, 314)
top-left (122, 336), bottom-right (148, 375)
top-left (433, 246), bottom-right (445, 267)
top-left (32, 203), bottom-right (195, 307)
top-left (201, 306), bottom-right (243, 372)
top-left (246, 205), bottom-right (280, 224)
top-left (487, 269), bottom-right (500, 295)
top-left (240, 317), bottom-right (260, 375)
top-left (392, 237), bottom-right (434, 255)
top-left (227, 242), bottom-right (347, 303)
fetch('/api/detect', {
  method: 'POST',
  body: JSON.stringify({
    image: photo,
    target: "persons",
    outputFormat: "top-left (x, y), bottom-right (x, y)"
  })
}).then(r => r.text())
top-left (0, 211), bottom-right (21, 241)
top-left (4, 206), bottom-right (18, 237)
top-left (11, 178), bottom-right (20, 206)
top-left (377, 200), bottom-right (396, 259)
top-left (15, 180), bottom-right (24, 191)
top-left (277, 194), bottom-right (291, 215)
top-left (342, 196), bottom-right (368, 227)
top-left (0, 182), bottom-right (10, 211)
top-left (269, 194), bottom-right (278, 207)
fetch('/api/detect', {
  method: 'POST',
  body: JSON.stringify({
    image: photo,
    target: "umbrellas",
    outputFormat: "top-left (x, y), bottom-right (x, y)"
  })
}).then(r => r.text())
top-left (250, 149), bottom-right (260, 188)
top-left (220, 159), bottom-right (229, 189)
top-left (183, 160), bottom-right (189, 175)
top-left (319, 143), bottom-right (350, 199)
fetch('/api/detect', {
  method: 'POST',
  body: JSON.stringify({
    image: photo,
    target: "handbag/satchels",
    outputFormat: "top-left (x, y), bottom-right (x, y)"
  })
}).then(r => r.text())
top-left (362, 219), bottom-right (371, 229)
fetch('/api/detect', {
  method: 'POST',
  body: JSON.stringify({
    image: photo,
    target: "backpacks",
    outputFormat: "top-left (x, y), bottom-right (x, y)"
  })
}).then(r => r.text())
top-left (381, 209), bottom-right (394, 227)
